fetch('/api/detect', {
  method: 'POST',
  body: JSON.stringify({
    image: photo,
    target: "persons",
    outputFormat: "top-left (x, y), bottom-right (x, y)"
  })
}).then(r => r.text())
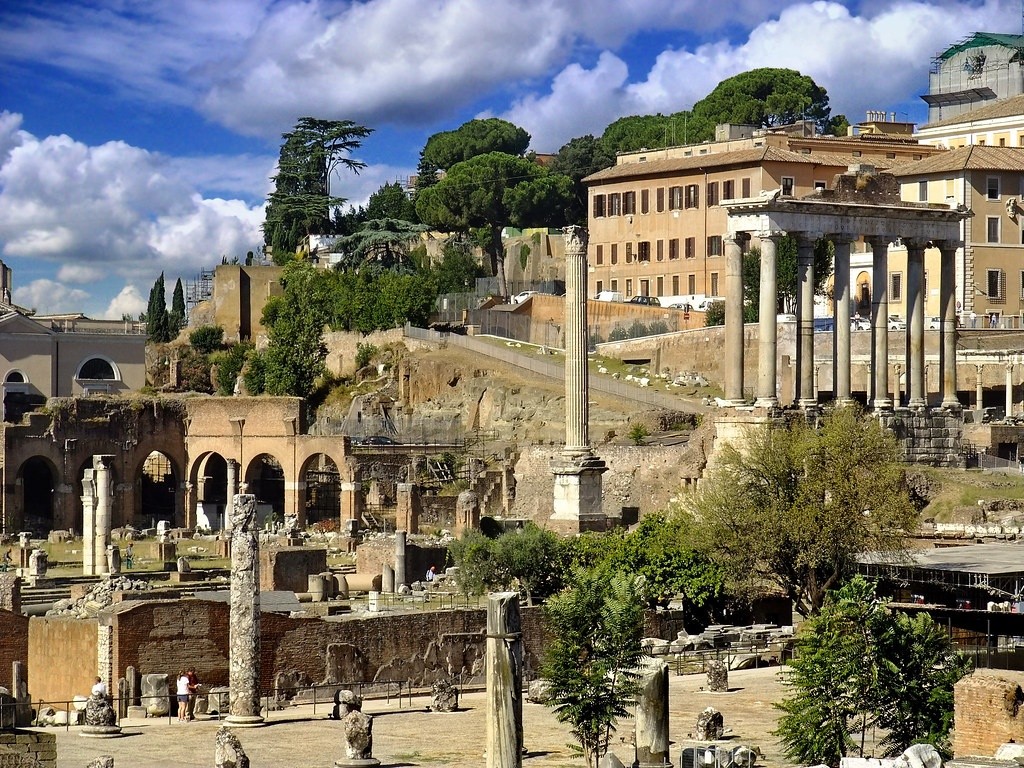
top-left (91, 676), bottom-right (107, 699)
top-left (176, 667), bottom-right (201, 722)
top-left (990, 313), bottom-right (998, 328)
top-left (969, 312), bottom-right (977, 327)
top-left (126, 543), bottom-right (134, 569)
top-left (426, 566), bottom-right (435, 582)
top-left (2, 548), bottom-right (12, 572)
top-left (853, 312), bottom-right (861, 331)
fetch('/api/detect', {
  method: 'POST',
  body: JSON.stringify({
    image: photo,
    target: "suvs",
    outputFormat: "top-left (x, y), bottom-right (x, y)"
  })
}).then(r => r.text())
top-left (623, 296), bottom-right (661, 306)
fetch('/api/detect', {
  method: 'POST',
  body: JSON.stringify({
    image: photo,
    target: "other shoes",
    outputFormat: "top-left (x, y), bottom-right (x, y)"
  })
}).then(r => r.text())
top-left (177, 718), bottom-right (185, 722)
top-left (191, 715), bottom-right (196, 720)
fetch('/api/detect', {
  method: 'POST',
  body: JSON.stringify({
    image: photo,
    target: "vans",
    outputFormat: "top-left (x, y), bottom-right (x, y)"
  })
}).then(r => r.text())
top-left (593, 291), bottom-right (623, 305)
top-left (513, 291), bottom-right (538, 304)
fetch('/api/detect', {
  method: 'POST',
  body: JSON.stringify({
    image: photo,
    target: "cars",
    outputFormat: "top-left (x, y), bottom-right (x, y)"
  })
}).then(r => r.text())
top-left (888, 316), bottom-right (906, 331)
top-left (850, 315), bottom-right (871, 332)
top-left (667, 302), bottom-right (694, 311)
top-left (930, 316), bottom-right (941, 329)
